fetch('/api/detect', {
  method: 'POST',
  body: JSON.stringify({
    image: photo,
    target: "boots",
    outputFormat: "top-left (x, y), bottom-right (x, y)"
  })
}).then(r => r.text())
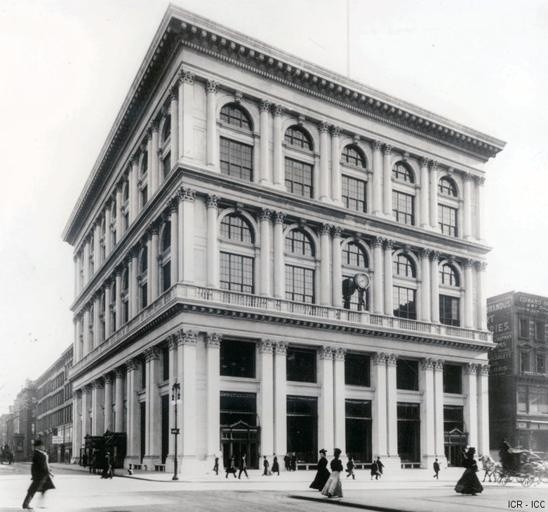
top-left (23, 493), bottom-right (33, 509)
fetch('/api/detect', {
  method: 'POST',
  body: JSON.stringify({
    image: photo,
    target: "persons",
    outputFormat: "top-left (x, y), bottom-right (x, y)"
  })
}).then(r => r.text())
top-left (309, 446), bottom-right (385, 498)
top-left (454, 446), bottom-right (483, 496)
top-left (23, 440), bottom-right (56, 510)
top-left (100, 452), bottom-right (114, 480)
top-left (213, 448), bottom-right (297, 479)
top-left (433, 458), bottom-right (440, 478)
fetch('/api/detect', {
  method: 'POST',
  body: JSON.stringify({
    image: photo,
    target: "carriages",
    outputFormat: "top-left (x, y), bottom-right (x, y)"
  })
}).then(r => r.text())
top-left (479, 447), bottom-right (548, 488)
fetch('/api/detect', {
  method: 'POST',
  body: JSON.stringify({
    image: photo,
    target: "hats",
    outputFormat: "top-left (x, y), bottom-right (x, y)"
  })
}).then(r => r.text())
top-left (33, 440), bottom-right (43, 446)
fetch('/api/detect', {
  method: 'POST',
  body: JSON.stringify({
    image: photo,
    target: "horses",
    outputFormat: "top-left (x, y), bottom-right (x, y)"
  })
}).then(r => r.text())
top-left (477, 453), bottom-right (503, 484)
